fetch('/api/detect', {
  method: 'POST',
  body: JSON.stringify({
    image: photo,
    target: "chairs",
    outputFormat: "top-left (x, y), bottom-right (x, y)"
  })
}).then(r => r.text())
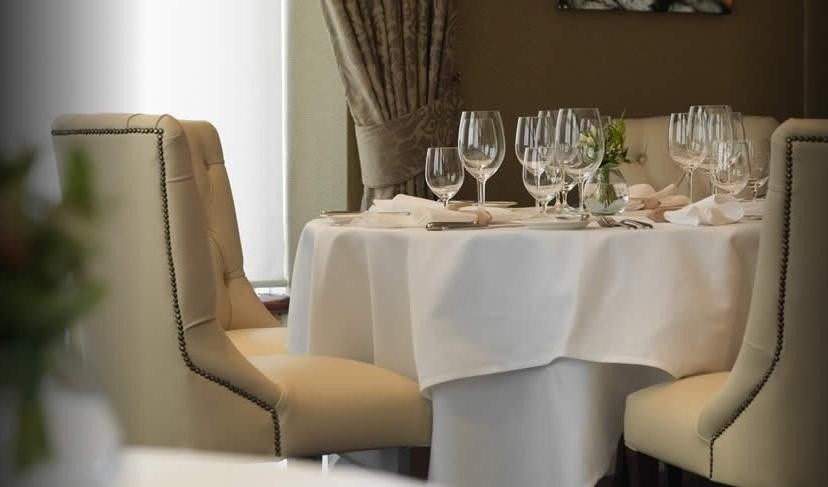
top-left (49, 112), bottom-right (433, 470)
top-left (578, 114), bottom-right (828, 485)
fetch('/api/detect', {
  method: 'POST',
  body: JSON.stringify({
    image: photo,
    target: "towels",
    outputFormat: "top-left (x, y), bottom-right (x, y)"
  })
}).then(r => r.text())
top-left (355, 190), bottom-right (476, 222)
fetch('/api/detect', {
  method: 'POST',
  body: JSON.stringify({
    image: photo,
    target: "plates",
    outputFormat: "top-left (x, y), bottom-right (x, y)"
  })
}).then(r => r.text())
top-left (524, 222), bottom-right (589, 229)
top-left (325, 214), bottom-right (361, 225)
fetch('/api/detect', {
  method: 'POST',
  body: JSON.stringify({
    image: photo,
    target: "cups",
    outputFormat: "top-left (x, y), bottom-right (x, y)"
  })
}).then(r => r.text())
top-left (582, 169), bottom-right (631, 214)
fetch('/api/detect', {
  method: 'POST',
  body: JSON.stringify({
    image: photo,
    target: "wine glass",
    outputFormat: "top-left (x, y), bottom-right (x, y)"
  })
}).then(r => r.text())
top-left (715, 112), bottom-right (764, 215)
top-left (672, 112), bottom-right (703, 221)
top-left (690, 104), bottom-right (734, 212)
top-left (459, 109), bottom-right (507, 220)
top-left (424, 144), bottom-right (463, 214)
top-left (513, 106), bottom-right (606, 216)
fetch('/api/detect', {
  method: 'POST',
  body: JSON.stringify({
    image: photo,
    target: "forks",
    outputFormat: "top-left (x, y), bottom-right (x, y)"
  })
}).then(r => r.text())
top-left (597, 216), bottom-right (653, 229)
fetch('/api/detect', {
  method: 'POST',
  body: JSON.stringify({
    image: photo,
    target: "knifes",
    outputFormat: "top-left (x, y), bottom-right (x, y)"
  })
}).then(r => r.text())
top-left (426, 221), bottom-right (524, 232)
